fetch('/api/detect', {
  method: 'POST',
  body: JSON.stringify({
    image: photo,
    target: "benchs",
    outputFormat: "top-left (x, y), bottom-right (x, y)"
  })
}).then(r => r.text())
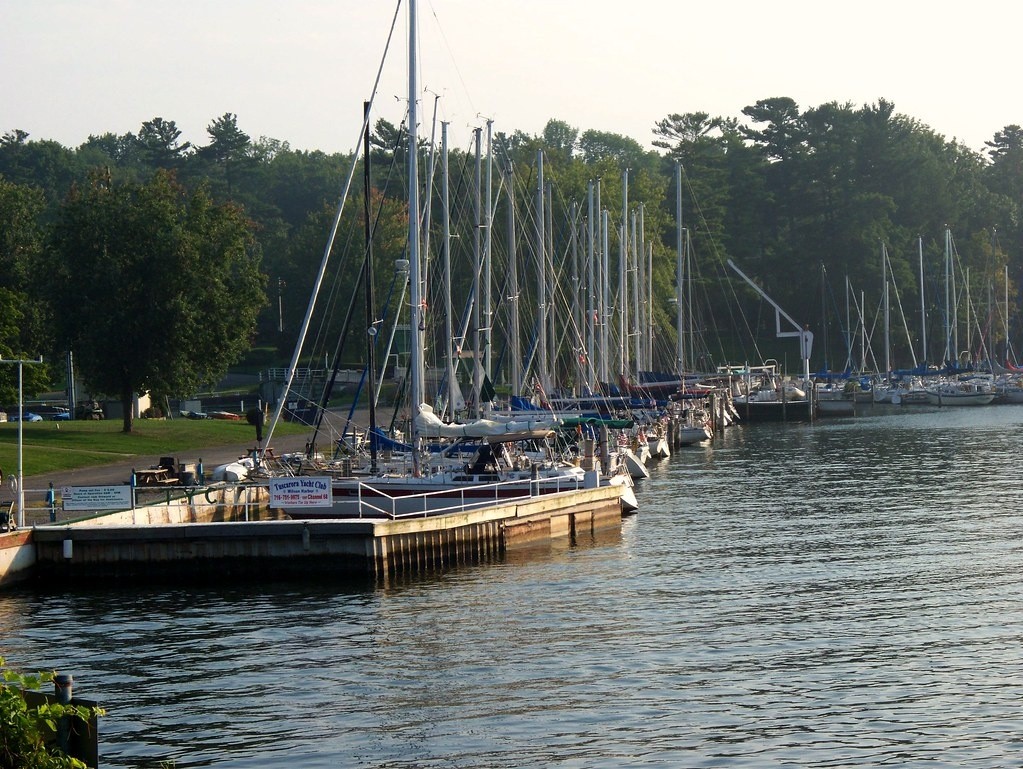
top-left (157, 478), bottom-right (179, 483)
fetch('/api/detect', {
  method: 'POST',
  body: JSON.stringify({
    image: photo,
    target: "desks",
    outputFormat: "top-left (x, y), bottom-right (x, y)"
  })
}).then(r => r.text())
top-left (136, 469), bottom-right (168, 487)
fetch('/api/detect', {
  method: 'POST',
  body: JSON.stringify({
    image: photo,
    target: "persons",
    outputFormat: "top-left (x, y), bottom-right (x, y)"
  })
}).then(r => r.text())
top-left (0, 470), bottom-right (14, 534)
top-left (567, 441), bottom-right (581, 455)
top-left (704, 397), bottom-right (709, 408)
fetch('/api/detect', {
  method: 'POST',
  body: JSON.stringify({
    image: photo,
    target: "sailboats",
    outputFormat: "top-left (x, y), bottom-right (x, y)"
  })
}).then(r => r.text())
top-left (242, 0), bottom-right (1023, 515)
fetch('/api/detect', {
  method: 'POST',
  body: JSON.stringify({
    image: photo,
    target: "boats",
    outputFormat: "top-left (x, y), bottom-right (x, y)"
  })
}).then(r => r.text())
top-left (9, 411), bottom-right (43, 423)
top-left (211, 457), bottom-right (264, 481)
top-left (207, 411), bottom-right (239, 420)
top-left (180, 409), bottom-right (208, 420)
